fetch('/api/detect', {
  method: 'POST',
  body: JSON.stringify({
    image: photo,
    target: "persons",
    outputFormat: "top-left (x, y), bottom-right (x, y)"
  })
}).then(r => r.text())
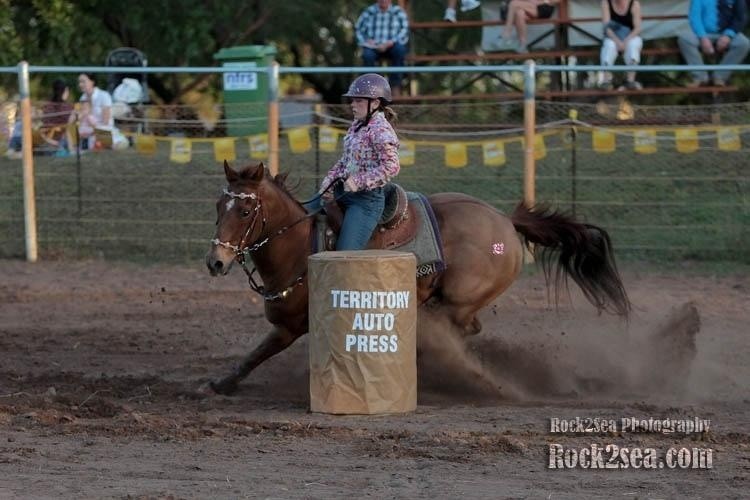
top-left (596, 0), bottom-right (645, 91)
top-left (353, 0), bottom-right (411, 98)
top-left (675, 0), bottom-right (750, 87)
top-left (496, 0), bottom-right (556, 54)
top-left (52, 74), bottom-right (117, 156)
top-left (312, 72), bottom-right (402, 252)
top-left (443, 0), bottom-right (482, 23)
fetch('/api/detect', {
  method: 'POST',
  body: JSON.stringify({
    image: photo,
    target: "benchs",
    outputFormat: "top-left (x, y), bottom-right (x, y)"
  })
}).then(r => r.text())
top-left (382, 1), bottom-right (739, 105)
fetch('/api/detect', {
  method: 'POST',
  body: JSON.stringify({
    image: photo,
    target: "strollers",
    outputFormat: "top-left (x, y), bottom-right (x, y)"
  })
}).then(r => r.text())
top-left (100, 48), bottom-right (149, 137)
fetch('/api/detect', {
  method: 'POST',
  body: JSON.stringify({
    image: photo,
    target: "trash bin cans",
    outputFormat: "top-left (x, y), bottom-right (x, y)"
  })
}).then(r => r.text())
top-left (213, 45), bottom-right (278, 137)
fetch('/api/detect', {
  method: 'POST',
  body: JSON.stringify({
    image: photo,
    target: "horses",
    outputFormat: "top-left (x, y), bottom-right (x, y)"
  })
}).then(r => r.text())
top-left (206, 161), bottom-right (632, 395)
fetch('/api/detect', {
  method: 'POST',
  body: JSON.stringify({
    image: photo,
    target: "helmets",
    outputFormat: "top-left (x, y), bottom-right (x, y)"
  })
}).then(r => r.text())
top-left (341, 72), bottom-right (393, 104)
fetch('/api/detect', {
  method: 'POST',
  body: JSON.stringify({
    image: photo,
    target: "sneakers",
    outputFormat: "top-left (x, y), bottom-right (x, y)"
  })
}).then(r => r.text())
top-left (600, 79), bottom-right (727, 91)
top-left (443, 0), bottom-right (482, 22)
